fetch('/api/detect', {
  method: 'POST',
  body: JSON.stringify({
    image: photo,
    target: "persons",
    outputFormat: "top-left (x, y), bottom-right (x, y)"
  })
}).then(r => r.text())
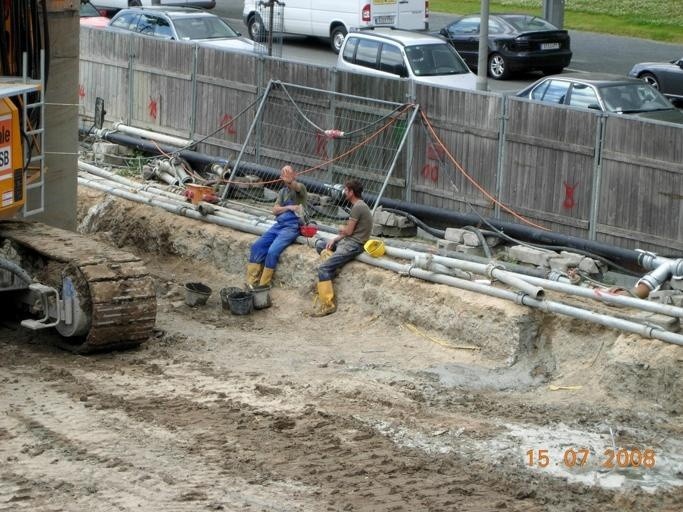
top-left (310, 179), bottom-right (372, 317)
top-left (244, 165), bottom-right (306, 291)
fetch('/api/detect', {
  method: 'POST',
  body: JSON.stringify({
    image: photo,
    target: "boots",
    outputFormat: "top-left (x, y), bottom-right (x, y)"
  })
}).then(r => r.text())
top-left (320, 249), bottom-right (341, 275)
top-left (247, 263), bottom-right (274, 287)
top-left (311, 280), bottom-right (335, 317)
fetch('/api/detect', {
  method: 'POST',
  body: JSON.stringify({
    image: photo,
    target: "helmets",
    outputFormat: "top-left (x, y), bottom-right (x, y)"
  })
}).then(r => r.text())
top-left (364, 240), bottom-right (385, 256)
top-left (301, 226), bottom-right (316, 237)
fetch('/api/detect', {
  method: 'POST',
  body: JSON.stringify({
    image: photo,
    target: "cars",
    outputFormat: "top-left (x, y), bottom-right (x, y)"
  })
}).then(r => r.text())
top-left (106, 6), bottom-right (270, 56)
top-left (628, 58), bottom-right (682, 108)
top-left (79, 1), bottom-right (110, 30)
top-left (88, 0), bottom-right (217, 16)
top-left (427, 12), bottom-right (573, 81)
top-left (510, 72), bottom-right (682, 127)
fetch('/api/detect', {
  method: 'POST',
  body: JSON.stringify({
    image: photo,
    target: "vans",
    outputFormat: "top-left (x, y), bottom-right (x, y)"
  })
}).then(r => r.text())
top-left (334, 30), bottom-right (491, 95)
top-left (242, 0), bottom-right (430, 56)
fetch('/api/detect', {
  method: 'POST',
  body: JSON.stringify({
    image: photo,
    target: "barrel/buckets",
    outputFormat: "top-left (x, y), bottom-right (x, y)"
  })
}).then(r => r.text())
top-left (225, 291), bottom-right (255, 315)
top-left (220, 286), bottom-right (244, 309)
top-left (184, 281), bottom-right (211, 307)
top-left (247, 283), bottom-right (272, 309)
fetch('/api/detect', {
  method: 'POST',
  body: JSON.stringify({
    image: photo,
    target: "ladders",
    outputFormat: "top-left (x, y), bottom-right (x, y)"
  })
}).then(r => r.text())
top-left (21, 50), bottom-right (45, 218)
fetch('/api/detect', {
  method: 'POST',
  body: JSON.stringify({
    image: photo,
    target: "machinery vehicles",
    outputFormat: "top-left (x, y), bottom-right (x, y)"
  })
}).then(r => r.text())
top-left (0, 51), bottom-right (158, 355)
